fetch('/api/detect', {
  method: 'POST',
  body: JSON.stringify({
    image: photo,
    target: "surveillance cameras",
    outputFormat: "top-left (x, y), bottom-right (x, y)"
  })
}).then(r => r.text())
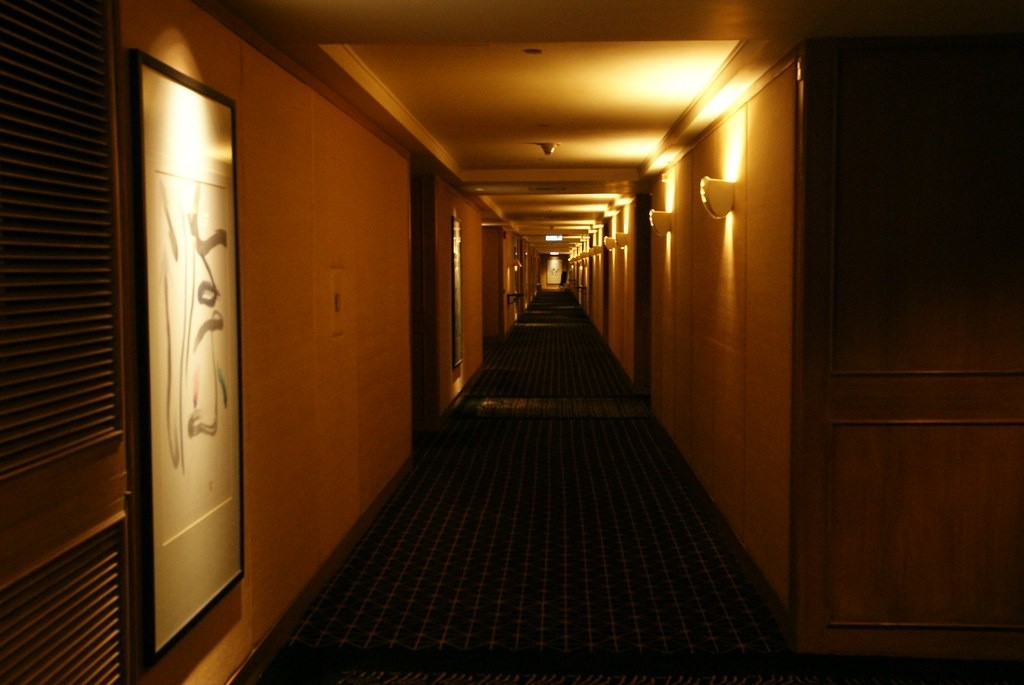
top-left (540, 143), bottom-right (556, 155)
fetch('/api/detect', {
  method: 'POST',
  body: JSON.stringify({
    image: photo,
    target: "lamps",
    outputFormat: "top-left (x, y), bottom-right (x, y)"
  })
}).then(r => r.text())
top-left (568, 233), bottom-right (626, 263)
top-left (699, 175), bottom-right (735, 219)
top-left (649, 209), bottom-right (672, 236)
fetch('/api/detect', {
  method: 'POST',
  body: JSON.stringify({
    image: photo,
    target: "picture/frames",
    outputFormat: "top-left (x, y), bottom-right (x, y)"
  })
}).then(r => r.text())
top-left (127, 47), bottom-right (244, 673)
